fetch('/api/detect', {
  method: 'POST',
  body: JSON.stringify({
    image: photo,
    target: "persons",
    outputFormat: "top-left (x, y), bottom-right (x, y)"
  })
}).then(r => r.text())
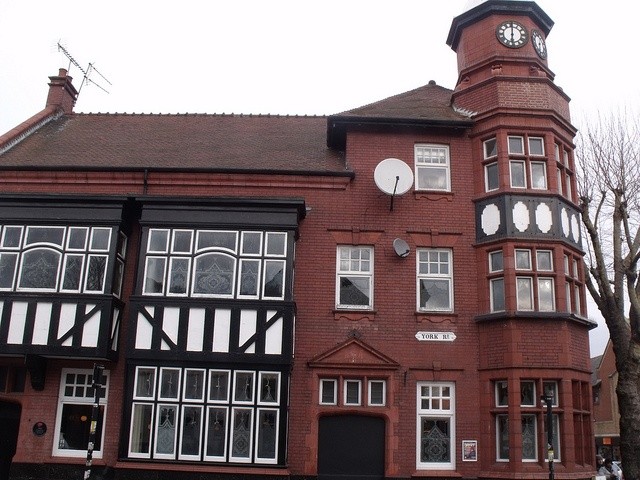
top-left (599, 458), bottom-right (619, 479)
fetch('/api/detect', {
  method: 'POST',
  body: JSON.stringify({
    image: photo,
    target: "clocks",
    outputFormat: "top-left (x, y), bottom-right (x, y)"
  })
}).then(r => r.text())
top-left (495, 20), bottom-right (529, 48)
top-left (531, 29), bottom-right (547, 60)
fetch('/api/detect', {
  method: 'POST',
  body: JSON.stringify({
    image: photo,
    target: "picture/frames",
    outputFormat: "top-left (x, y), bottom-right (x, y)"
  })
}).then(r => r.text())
top-left (462, 440), bottom-right (477, 462)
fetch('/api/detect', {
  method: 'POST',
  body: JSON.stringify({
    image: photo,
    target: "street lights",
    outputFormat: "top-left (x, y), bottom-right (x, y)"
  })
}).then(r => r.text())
top-left (545, 385), bottom-right (554, 480)
